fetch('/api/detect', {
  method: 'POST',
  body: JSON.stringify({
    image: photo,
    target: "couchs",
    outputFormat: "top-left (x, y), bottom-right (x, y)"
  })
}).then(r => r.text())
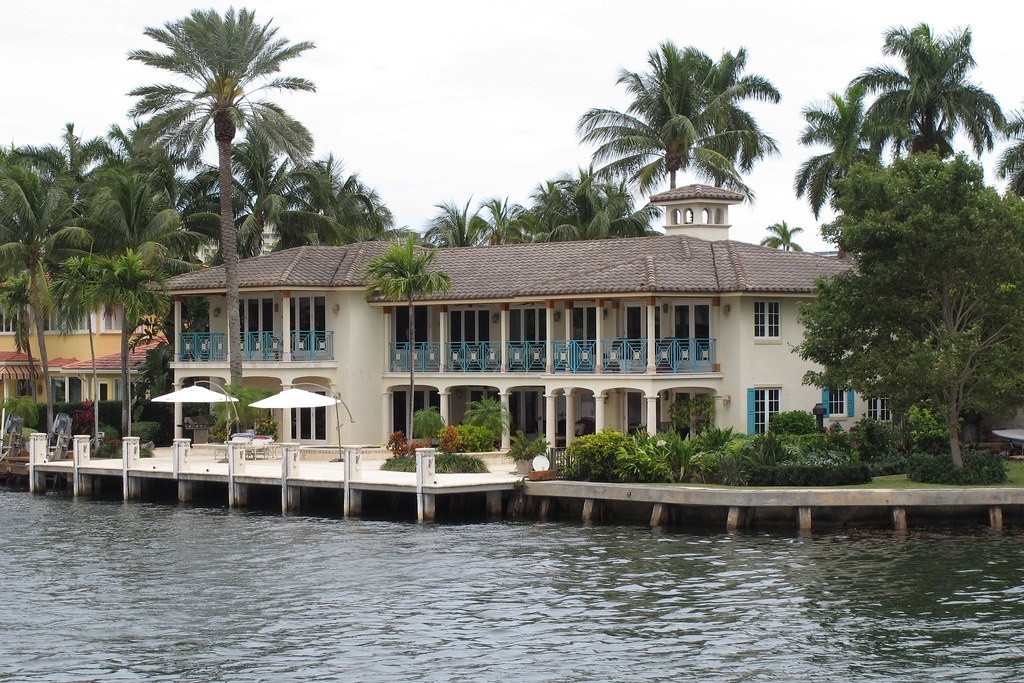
top-left (603, 338), bottom-right (635, 370)
top-left (656, 337), bottom-right (676, 370)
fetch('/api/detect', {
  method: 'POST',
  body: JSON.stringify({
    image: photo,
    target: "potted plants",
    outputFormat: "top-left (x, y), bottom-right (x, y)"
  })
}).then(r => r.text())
top-left (505, 430), bottom-right (549, 474)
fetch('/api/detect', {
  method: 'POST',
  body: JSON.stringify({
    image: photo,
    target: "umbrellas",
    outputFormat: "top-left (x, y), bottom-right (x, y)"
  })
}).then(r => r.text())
top-left (151, 380), bottom-right (239, 463)
top-left (247, 382), bottom-right (354, 461)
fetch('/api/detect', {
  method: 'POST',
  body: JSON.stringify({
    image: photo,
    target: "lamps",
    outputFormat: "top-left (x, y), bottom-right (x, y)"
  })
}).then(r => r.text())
top-left (528, 455), bottom-right (556, 481)
top-left (663, 303), bottom-right (669, 313)
top-left (332, 303), bottom-right (340, 313)
top-left (603, 309), bottom-right (608, 319)
top-left (723, 303), bottom-right (731, 316)
top-left (213, 308), bottom-right (221, 318)
top-left (492, 312), bottom-right (499, 324)
top-left (553, 310), bottom-right (562, 321)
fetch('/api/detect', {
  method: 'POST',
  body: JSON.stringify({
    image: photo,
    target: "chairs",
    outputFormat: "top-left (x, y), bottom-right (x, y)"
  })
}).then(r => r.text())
top-left (214, 433), bottom-right (275, 462)
